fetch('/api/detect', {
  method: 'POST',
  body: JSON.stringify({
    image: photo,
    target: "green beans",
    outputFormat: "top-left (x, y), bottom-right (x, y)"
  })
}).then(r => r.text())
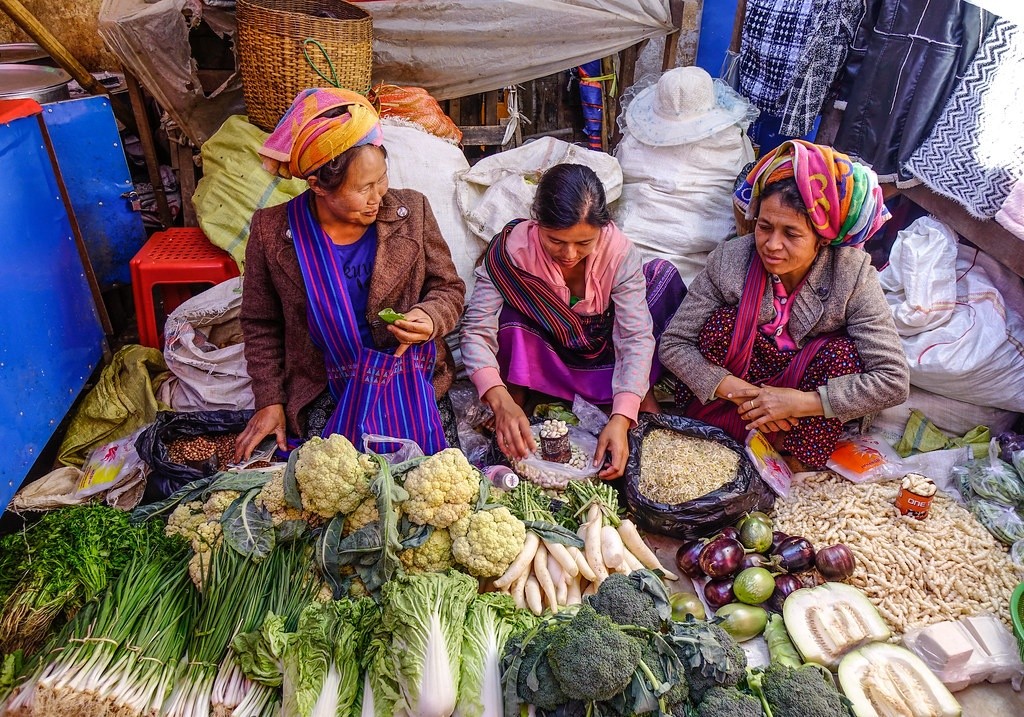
top-left (952, 457), bottom-right (1024, 545)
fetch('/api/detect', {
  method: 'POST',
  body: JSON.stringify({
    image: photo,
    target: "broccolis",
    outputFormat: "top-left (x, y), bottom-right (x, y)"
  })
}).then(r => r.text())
top-left (166, 432), bottom-right (527, 608)
top-left (501, 571), bottom-right (854, 717)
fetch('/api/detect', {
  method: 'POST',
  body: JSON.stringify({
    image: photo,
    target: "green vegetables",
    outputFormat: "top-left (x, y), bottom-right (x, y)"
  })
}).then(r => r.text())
top-left (1, 500), bottom-right (164, 657)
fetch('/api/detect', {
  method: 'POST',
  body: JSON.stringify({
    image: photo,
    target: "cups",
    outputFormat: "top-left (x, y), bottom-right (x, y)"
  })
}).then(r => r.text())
top-left (539, 429), bottom-right (572, 464)
top-left (894, 473), bottom-right (937, 520)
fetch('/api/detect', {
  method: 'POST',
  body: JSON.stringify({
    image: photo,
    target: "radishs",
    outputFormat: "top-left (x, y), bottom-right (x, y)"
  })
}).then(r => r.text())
top-left (493, 477), bottom-right (679, 617)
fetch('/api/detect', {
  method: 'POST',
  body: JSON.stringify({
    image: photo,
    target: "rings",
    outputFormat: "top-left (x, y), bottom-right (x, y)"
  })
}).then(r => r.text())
top-left (406, 340), bottom-right (409, 344)
top-left (750, 399), bottom-right (755, 409)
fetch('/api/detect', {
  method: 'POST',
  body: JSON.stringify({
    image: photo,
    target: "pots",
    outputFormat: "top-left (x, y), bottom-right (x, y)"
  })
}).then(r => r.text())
top-left (0, 43), bottom-right (54, 67)
top-left (0, 63), bottom-right (70, 104)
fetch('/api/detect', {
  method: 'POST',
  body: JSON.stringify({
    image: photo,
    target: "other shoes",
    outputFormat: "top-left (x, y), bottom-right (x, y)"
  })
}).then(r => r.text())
top-left (481, 392), bottom-right (529, 442)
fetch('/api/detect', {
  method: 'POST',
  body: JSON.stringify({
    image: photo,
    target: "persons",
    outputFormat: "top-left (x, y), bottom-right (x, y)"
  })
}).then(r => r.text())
top-left (458, 162), bottom-right (688, 481)
top-left (657, 138), bottom-right (912, 472)
top-left (233, 84), bottom-right (467, 464)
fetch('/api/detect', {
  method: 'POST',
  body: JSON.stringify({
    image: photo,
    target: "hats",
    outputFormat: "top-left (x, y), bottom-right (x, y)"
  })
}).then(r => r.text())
top-left (616, 65), bottom-right (753, 148)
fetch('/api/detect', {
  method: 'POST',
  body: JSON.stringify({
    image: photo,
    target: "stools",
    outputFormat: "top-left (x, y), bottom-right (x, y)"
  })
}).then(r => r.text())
top-left (128, 227), bottom-right (240, 352)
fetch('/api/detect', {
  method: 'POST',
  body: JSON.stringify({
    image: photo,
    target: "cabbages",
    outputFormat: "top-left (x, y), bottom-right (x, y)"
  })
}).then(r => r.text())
top-left (226, 567), bottom-right (540, 717)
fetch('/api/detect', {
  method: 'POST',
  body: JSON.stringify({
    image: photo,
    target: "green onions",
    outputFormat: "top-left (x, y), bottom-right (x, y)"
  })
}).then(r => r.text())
top-left (0, 534), bottom-right (324, 717)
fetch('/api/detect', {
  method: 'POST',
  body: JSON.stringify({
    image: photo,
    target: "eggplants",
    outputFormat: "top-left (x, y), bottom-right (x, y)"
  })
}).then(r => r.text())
top-left (668, 509), bottom-right (856, 643)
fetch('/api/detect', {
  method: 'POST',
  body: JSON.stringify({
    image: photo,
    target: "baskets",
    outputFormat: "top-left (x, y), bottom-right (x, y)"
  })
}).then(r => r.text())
top-left (235, 0), bottom-right (373, 134)
